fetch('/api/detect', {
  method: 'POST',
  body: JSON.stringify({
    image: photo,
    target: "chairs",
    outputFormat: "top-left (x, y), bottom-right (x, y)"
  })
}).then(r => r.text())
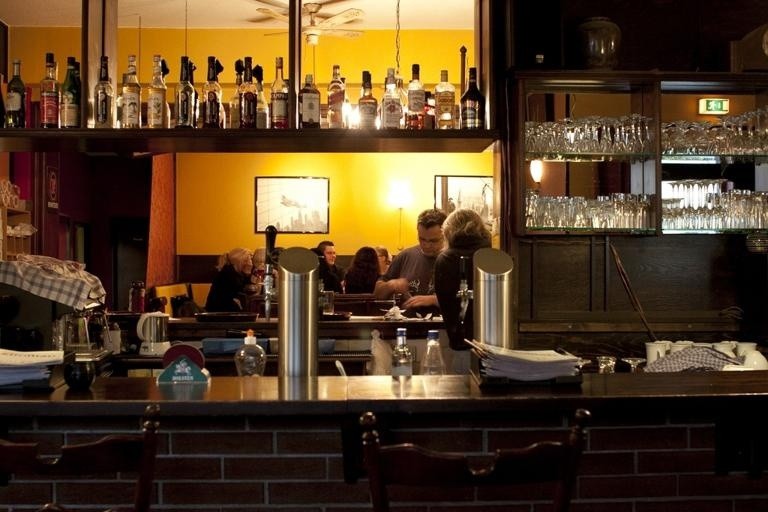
top-left (154, 283), bottom-right (190, 319)
top-left (190, 283), bottom-right (213, 312)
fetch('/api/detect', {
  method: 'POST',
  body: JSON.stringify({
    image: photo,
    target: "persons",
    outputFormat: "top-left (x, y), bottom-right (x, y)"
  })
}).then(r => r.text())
top-left (373, 246), bottom-right (391, 278)
top-left (371, 207), bottom-right (448, 317)
top-left (432, 207), bottom-right (494, 374)
top-left (344, 246), bottom-right (381, 295)
top-left (204, 240), bottom-right (346, 312)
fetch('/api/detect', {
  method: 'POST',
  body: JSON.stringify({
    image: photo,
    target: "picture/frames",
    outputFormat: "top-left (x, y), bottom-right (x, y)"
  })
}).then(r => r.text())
top-left (434, 176), bottom-right (493, 237)
top-left (253, 177), bottom-right (330, 234)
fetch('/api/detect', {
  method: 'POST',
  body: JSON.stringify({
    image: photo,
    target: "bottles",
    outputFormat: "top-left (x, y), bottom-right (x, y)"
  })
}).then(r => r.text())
top-left (40, 53), bottom-right (81, 129)
top-left (270, 57), bottom-right (290, 129)
top-left (5, 58), bottom-right (32, 130)
top-left (405, 64), bottom-right (433, 129)
top-left (298, 74), bottom-right (322, 130)
top-left (119, 54), bottom-right (142, 128)
top-left (357, 71), bottom-right (377, 129)
top-left (435, 69), bottom-right (456, 129)
top-left (327, 65), bottom-right (351, 129)
top-left (147, 55), bottom-right (171, 129)
top-left (175, 56), bottom-right (199, 129)
top-left (108, 322), bottom-right (121, 355)
top-left (202, 56), bottom-right (226, 129)
top-left (389, 328), bottom-right (413, 376)
top-left (460, 67), bottom-right (483, 130)
top-left (420, 330), bottom-right (448, 376)
top-left (93, 56), bottom-right (114, 128)
top-left (128, 280), bottom-right (147, 313)
top-left (230, 57), bottom-right (269, 129)
top-left (233, 329), bottom-right (267, 376)
top-left (379, 68), bottom-right (405, 130)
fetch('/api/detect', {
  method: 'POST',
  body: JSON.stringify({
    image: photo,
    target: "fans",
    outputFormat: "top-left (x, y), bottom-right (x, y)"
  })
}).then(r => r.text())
top-left (256, 4), bottom-right (365, 45)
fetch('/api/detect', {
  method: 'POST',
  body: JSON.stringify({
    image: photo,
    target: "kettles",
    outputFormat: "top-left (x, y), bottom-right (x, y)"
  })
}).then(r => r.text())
top-left (137, 311), bottom-right (171, 358)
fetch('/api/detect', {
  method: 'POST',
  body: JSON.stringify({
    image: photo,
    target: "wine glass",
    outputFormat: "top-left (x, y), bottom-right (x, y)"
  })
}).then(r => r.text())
top-left (621, 357), bottom-right (648, 372)
top-left (661, 111), bottom-right (768, 155)
top-left (526, 113), bottom-right (656, 153)
top-left (660, 179), bottom-right (768, 229)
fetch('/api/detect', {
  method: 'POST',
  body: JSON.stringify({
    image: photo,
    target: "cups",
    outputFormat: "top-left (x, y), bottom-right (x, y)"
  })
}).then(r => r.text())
top-left (597, 356), bottom-right (617, 371)
top-left (323, 291), bottom-right (334, 315)
top-left (644, 340), bottom-right (768, 371)
top-left (525, 189), bottom-right (652, 229)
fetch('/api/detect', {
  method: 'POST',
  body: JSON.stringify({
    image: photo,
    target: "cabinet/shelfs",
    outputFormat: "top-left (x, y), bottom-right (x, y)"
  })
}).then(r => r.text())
top-left (495, 1), bottom-right (768, 323)
